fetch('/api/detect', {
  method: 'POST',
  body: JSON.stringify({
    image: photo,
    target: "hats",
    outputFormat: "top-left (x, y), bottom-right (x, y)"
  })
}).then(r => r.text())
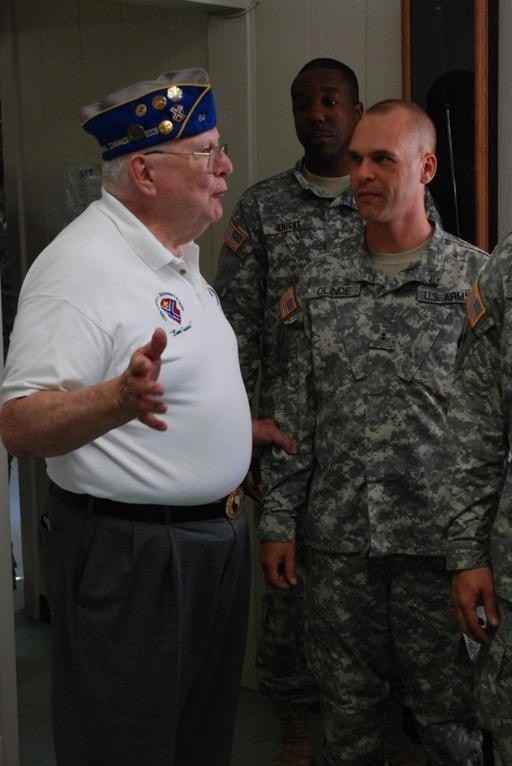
top-left (79, 68), bottom-right (216, 161)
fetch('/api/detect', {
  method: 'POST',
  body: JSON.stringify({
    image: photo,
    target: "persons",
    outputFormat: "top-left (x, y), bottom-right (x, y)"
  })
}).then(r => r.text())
top-left (0, 67), bottom-right (304, 766)
top-left (422, 229), bottom-right (512, 766)
top-left (210, 55), bottom-right (446, 765)
top-left (253, 95), bottom-right (494, 766)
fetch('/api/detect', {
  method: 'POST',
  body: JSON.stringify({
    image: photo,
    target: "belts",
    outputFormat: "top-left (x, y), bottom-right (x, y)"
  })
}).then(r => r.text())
top-left (50, 478), bottom-right (244, 525)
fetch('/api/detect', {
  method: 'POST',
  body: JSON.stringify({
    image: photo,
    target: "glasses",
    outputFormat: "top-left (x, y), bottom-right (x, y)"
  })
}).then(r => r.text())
top-left (143, 144), bottom-right (228, 174)
